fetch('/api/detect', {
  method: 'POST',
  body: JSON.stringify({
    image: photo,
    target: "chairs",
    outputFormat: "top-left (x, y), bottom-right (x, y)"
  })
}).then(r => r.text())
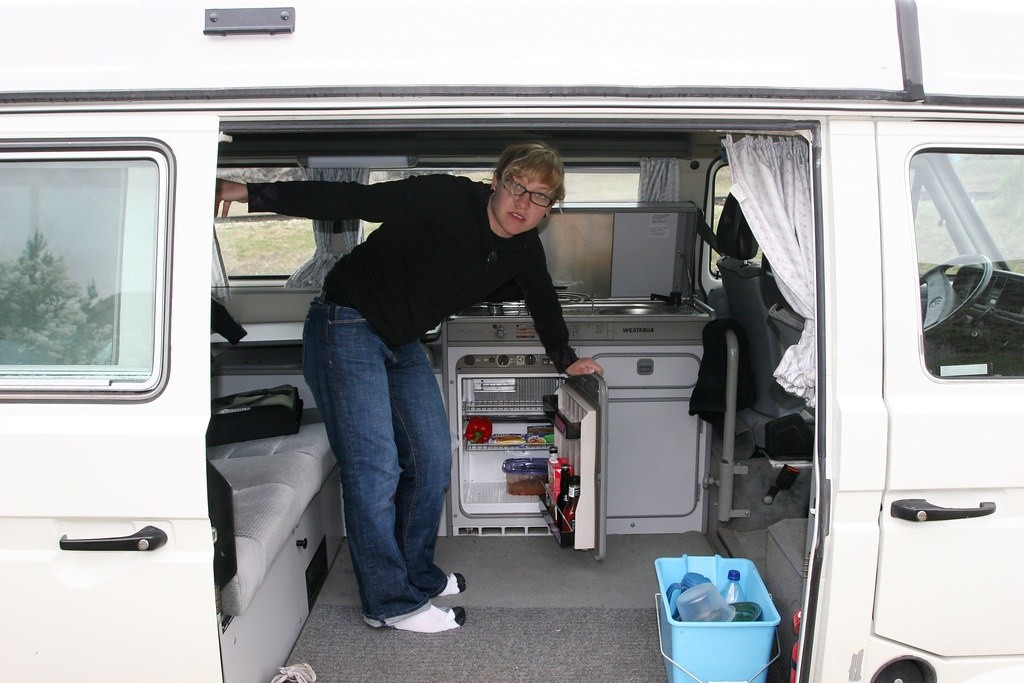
top-left (718, 193), bottom-right (805, 418)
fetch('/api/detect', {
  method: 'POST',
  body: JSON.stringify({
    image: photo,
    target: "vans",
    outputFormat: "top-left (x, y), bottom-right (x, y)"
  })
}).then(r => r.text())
top-left (1, 1), bottom-right (1024, 683)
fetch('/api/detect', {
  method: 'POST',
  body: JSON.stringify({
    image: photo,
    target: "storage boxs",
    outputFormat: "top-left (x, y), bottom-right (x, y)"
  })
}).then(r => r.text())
top-left (206, 385), bottom-right (303, 447)
top-left (652, 552), bottom-right (782, 683)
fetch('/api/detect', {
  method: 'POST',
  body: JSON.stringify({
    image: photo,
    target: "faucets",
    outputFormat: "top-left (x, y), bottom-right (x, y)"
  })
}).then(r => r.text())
top-left (650, 291), bottom-right (682, 307)
top-left (677, 249), bottom-right (698, 307)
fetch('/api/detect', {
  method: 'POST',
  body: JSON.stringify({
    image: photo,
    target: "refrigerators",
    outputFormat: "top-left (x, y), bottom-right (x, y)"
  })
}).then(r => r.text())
top-left (458, 352), bottom-right (615, 566)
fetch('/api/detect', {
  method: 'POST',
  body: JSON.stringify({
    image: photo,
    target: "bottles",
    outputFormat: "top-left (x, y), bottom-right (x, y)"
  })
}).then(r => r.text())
top-left (718, 570), bottom-right (747, 606)
top-left (561, 476), bottom-right (581, 548)
top-left (557, 465), bottom-right (573, 531)
top-left (548, 447), bottom-right (559, 492)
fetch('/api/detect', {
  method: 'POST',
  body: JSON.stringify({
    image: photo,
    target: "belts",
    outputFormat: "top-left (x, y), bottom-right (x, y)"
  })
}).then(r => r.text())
top-left (319, 290), bottom-right (346, 307)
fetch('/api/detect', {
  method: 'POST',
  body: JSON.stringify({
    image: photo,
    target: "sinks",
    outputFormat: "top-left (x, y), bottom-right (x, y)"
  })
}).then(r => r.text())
top-left (600, 306), bottom-right (693, 315)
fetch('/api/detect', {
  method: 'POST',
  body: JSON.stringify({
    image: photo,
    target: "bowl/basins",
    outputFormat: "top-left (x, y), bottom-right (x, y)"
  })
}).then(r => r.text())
top-left (676, 581), bottom-right (737, 627)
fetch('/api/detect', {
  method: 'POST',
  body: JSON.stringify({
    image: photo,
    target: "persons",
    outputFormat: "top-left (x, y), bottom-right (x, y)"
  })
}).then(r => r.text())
top-left (212, 138), bottom-right (607, 637)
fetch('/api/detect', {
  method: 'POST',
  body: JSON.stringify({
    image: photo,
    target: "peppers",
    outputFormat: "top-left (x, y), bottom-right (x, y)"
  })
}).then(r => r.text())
top-left (465, 416), bottom-right (492, 443)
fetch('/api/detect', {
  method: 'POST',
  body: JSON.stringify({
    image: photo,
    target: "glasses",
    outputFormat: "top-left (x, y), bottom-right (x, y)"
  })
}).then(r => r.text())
top-left (497, 176), bottom-right (555, 208)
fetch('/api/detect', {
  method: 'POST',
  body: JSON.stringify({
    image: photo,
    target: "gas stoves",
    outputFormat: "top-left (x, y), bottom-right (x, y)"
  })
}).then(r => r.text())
top-left (441, 284), bottom-right (716, 344)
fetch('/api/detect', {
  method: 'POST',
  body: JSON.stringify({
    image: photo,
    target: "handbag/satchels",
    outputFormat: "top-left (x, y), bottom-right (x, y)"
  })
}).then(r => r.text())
top-left (205, 384), bottom-right (304, 447)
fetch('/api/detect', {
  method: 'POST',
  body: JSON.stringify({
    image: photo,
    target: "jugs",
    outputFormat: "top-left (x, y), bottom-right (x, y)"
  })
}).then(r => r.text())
top-left (665, 572), bottom-right (712, 621)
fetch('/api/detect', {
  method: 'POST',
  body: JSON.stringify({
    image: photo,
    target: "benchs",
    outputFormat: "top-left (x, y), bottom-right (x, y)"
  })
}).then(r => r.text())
top-left (206, 423), bottom-right (345, 683)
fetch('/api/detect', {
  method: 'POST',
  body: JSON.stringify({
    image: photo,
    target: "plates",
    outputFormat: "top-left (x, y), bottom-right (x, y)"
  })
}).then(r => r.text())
top-left (725, 600), bottom-right (762, 623)
top-left (491, 435), bottom-right (547, 445)
